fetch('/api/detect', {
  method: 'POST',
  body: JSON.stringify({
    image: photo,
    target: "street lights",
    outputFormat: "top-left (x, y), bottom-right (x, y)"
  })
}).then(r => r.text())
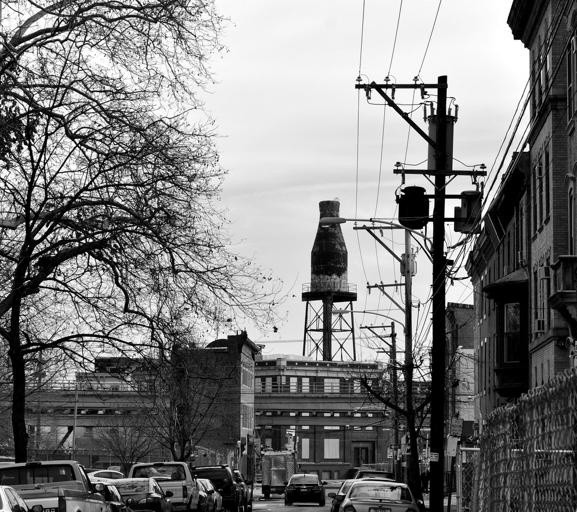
top-left (314, 214), bottom-right (446, 511)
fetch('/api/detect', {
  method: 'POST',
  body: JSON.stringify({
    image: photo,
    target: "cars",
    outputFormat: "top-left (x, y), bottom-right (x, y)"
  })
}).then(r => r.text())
top-left (282, 473), bottom-right (329, 508)
top-left (328, 467), bottom-right (424, 512)
top-left (0, 454), bottom-right (252, 511)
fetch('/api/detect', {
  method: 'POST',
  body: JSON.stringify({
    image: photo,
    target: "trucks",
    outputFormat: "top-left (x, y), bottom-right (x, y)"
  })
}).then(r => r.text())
top-left (259, 448), bottom-right (299, 499)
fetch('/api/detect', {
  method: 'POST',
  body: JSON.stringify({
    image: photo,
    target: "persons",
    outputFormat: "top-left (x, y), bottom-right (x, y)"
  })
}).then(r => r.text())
top-left (233, 469), bottom-right (246, 512)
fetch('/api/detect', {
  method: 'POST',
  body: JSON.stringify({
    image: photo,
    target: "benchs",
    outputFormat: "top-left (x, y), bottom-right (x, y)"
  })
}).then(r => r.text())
top-left (139, 470), bottom-right (184, 481)
